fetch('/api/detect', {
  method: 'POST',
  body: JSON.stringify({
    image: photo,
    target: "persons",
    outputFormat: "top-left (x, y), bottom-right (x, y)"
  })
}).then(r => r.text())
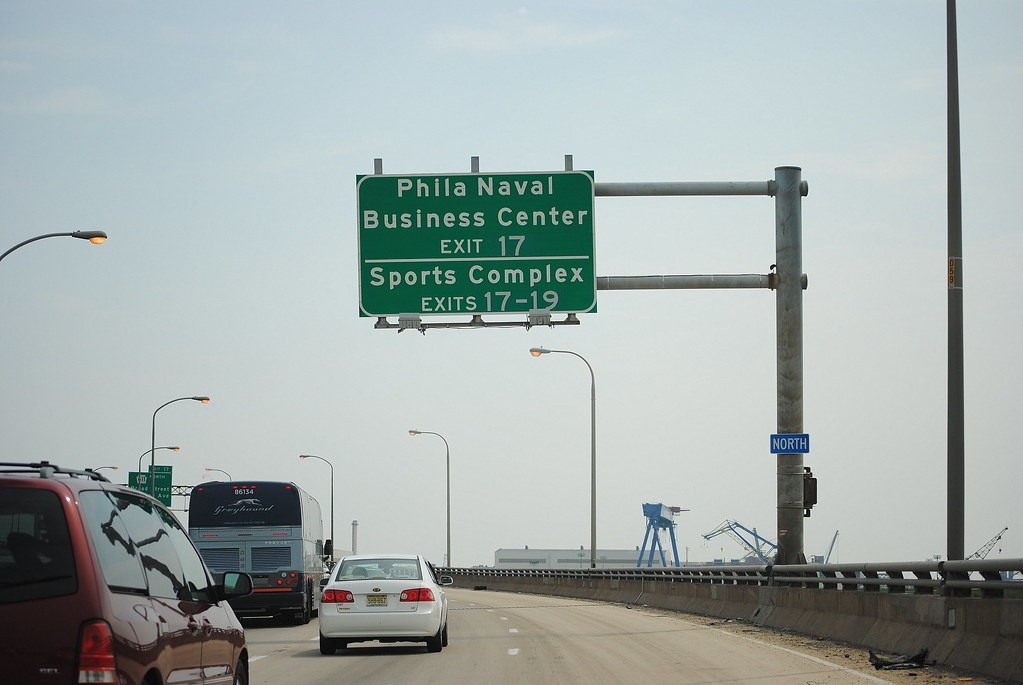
top-left (378, 562), bottom-right (393, 574)
top-left (353, 567), bottom-right (367, 577)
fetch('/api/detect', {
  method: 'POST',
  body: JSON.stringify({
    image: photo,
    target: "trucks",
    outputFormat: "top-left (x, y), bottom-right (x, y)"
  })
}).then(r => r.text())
top-left (188, 480), bottom-right (332, 626)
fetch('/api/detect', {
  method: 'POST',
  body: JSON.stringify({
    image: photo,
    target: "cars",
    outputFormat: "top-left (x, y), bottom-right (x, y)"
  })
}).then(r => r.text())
top-left (318, 553), bottom-right (453, 657)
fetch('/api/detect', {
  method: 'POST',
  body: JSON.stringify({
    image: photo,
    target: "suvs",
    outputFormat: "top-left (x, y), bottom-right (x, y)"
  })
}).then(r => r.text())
top-left (0, 460), bottom-right (254, 685)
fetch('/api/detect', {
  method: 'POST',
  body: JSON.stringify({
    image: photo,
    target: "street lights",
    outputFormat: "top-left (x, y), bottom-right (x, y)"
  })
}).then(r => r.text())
top-left (206, 469), bottom-right (231, 482)
top-left (409, 430), bottom-right (450, 569)
top-left (139, 446), bottom-right (180, 491)
top-left (531, 348), bottom-right (597, 570)
top-left (87, 466), bottom-right (118, 481)
top-left (299, 455), bottom-right (333, 561)
top-left (151, 397), bottom-right (210, 497)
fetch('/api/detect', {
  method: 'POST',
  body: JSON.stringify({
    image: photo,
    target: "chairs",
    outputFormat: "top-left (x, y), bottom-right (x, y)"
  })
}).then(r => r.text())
top-left (352, 567), bottom-right (367, 577)
